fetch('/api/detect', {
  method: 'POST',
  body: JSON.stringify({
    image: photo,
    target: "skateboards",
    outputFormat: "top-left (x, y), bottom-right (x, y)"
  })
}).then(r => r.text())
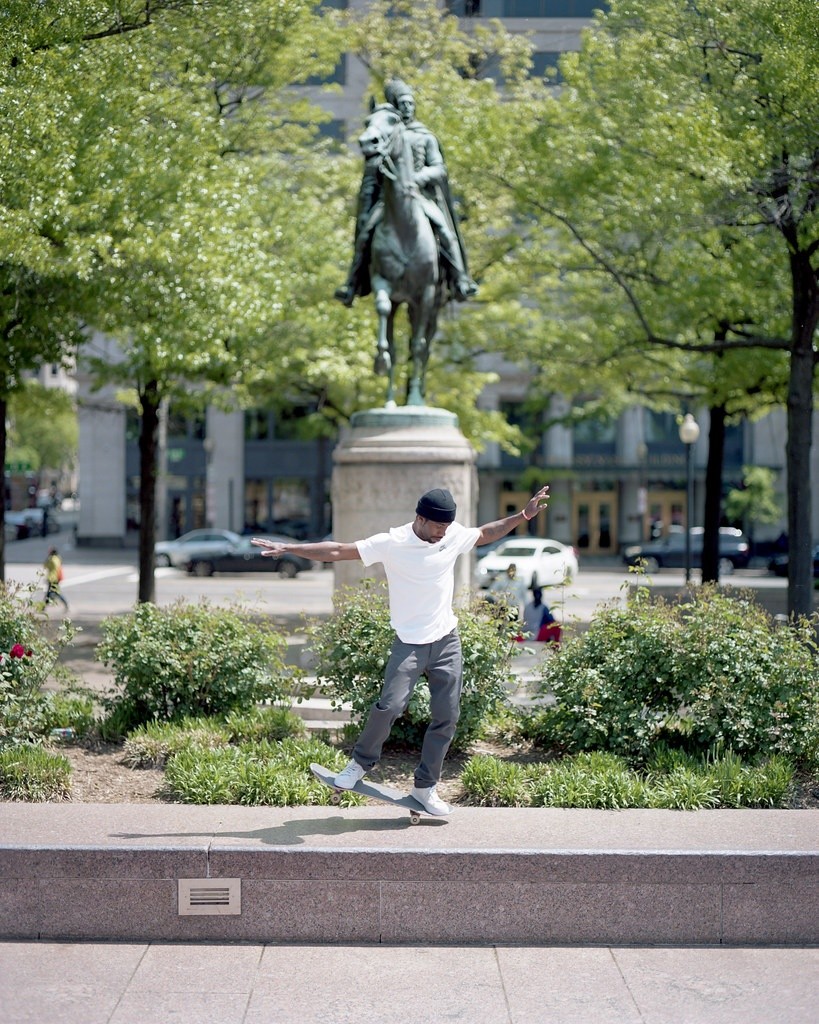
top-left (310, 762), bottom-right (455, 825)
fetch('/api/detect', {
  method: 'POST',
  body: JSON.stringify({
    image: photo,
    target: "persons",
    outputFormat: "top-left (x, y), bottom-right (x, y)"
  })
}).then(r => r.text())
top-left (514, 589), bottom-right (561, 652)
top-left (251, 486), bottom-right (550, 815)
top-left (39, 547), bottom-right (71, 615)
top-left (334, 78), bottom-right (478, 308)
top-left (485, 564), bottom-right (529, 630)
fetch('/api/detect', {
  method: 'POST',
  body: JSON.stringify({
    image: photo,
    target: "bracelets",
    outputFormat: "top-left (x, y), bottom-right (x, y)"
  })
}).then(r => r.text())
top-left (522, 508), bottom-right (531, 521)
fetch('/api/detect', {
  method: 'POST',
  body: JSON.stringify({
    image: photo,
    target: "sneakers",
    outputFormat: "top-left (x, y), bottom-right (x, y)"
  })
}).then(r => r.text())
top-left (410, 784), bottom-right (450, 816)
top-left (334, 757), bottom-right (367, 790)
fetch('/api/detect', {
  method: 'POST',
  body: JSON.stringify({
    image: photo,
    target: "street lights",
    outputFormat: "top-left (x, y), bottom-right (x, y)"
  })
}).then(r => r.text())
top-left (679, 413), bottom-right (700, 581)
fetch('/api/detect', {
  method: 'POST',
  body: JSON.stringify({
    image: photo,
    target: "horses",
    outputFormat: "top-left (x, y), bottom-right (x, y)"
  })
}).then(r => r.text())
top-left (356, 89), bottom-right (451, 409)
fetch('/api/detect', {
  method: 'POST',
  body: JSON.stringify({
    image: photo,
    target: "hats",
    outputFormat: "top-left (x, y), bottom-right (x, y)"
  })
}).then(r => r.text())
top-left (415, 488), bottom-right (458, 523)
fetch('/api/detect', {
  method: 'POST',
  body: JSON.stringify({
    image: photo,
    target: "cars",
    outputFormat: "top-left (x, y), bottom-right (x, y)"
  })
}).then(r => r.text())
top-left (154, 528), bottom-right (334, 579)
top-left (767, 545), bottom-right (819, 580)
top-left (474, 535), bottom-right (579, 590)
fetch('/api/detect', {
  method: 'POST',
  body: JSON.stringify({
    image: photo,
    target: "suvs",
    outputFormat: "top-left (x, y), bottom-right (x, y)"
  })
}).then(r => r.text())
top-left (622, 525), bottom-right (750, 575)
